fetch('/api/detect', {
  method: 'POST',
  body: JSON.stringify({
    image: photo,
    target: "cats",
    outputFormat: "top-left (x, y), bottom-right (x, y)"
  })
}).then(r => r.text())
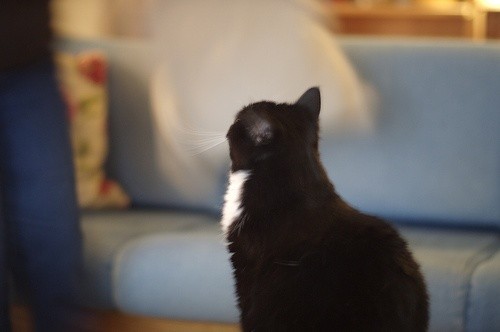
top-left (218, 86), bottom-right (430, 332)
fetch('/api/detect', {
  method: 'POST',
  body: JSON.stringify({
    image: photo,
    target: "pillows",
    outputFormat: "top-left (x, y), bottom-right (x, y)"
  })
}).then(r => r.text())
top-left (54, 50), bottom-right (127, 211)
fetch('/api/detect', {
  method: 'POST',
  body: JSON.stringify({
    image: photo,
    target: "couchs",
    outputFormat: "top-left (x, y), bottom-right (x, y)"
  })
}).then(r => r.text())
top-left (53, 28), bottom-right (499, 332)
